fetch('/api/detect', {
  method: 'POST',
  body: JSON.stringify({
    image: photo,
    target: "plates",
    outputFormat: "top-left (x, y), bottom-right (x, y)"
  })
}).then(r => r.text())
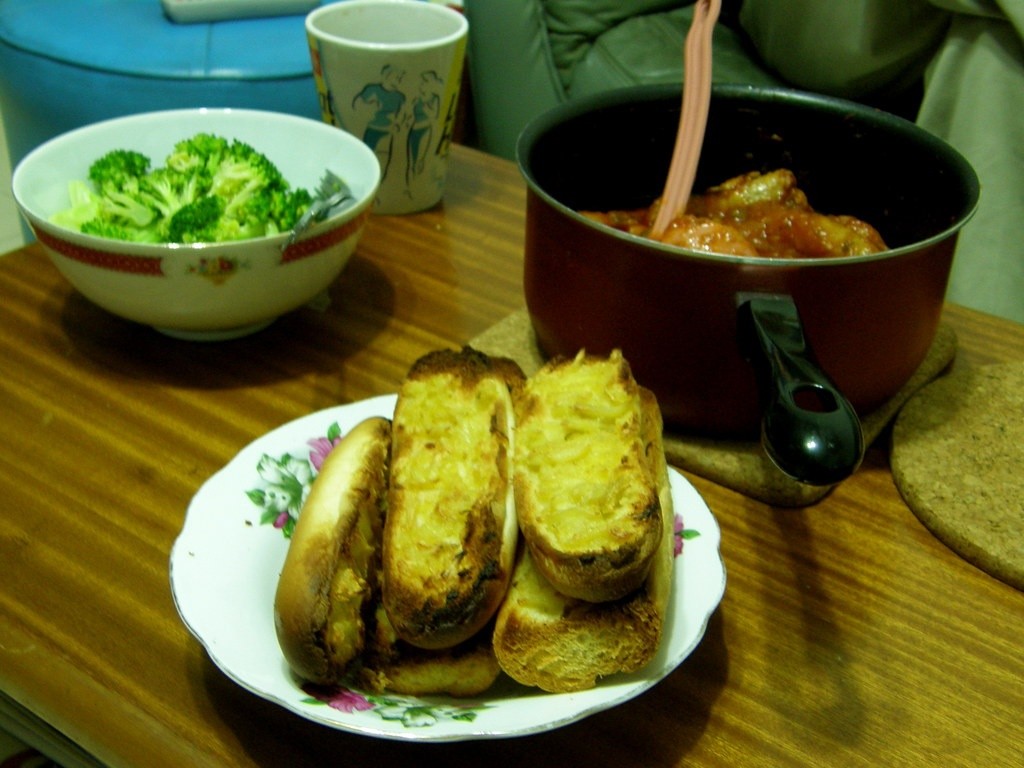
top-left (169, 393), bottom-right (726, 741)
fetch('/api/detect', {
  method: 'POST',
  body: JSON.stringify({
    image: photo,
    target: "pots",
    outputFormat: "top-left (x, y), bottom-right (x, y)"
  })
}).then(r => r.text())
top-left (514, 84), bottom-right (981, 487)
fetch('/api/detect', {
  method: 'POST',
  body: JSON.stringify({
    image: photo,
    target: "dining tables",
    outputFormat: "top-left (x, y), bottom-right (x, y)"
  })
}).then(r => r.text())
top-left (0, 143), bottom-right (1024, 767)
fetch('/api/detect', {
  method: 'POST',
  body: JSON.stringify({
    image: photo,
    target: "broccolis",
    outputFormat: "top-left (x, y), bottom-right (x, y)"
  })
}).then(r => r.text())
top-left (47, 131), bottom-right (330, 243)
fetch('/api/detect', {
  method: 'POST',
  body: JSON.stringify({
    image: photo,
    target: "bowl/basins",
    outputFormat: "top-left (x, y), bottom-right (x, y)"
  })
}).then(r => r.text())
top-left (11, 107), bottom-right (380, 340)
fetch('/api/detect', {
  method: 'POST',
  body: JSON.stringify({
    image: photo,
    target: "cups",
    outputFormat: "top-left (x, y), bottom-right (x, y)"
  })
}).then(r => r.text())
top-left (305, 0), bottom-right (469, 214)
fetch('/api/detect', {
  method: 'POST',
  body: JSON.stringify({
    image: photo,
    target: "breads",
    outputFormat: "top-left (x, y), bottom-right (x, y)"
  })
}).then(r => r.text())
top-left (271, 342), bottom-right (673, 699)
